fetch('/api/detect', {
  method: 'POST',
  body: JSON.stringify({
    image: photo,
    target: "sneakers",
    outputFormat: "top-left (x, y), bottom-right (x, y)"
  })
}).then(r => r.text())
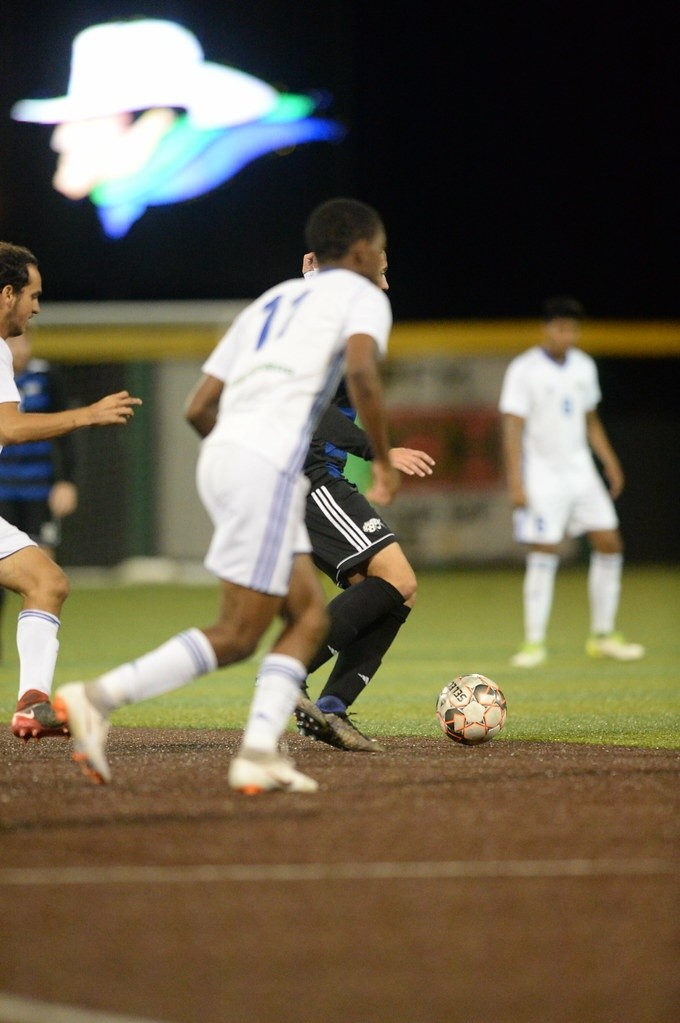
top-left (53, 682), bottom-right (111, 786)
top-left (309, 700), bottom-right (383, 752)
top-left (12, 688), bottom-right (70, 742)
top-left (228, 756), bottom-right (318, 796)
top-left (586, 631), bottom-right (646, 661)
top-left (294, 681), bottom-right (326, 733)
top-left (512, 641), bottom-right (548, 668)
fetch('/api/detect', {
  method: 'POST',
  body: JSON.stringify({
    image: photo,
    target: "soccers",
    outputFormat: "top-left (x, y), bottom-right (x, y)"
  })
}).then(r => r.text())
top-left (434, 673), bottom-right (508, 746)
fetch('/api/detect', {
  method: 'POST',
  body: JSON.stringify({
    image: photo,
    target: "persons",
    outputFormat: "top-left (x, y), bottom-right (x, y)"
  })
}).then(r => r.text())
top-left (52, 198), bottom-right (436, 793)
top-left (0, 242), bottom-right (141, 739)
top-left (497, 301), bottom-right (644, 667)
